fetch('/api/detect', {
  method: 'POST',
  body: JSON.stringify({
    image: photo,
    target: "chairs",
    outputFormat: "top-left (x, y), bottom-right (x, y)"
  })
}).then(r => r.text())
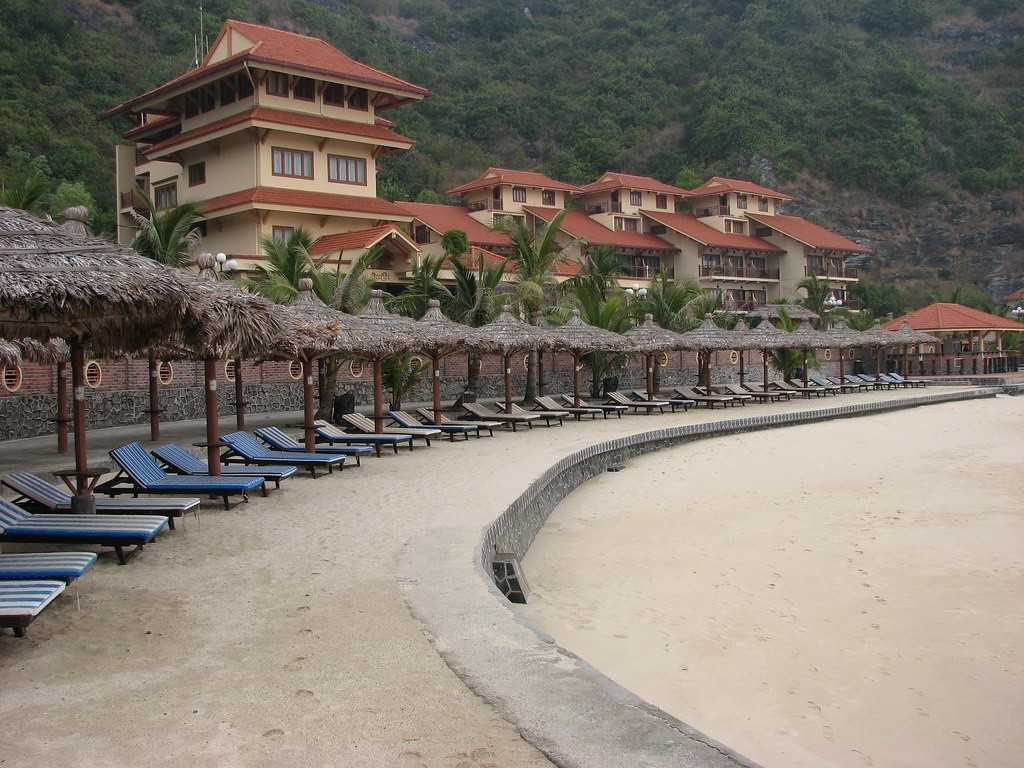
top-left (0, 373), bottom-right (931, 638)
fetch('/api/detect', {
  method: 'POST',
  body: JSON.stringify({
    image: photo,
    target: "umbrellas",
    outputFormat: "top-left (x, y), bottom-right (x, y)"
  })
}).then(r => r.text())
top-left (552, 309), bottom-right (626, 407)
top-left (825, 321), bottom-right (874, 392)
top-left (610, 313), bottom-right (704, 412)
top-left (419, 300), bottom-right (499, 425)
top-left (0, 206), bottom-right (193, 498)
top-left (892, 320), bottom-right (941, 389)
top-left (674, 314), bottom-right (754, 407)
top-left (356, 290), bottom-right (452, 458)
top-left (473, 304), bottom-right (557, 414)
top-left (160, 253), bottom-right (409, 479)
top-left (747, 320), bottom-right (809, 402)
top-left (860, 319), bottom-right (907, 389)
top-left (786, 321), bottom-right (839, 398)
